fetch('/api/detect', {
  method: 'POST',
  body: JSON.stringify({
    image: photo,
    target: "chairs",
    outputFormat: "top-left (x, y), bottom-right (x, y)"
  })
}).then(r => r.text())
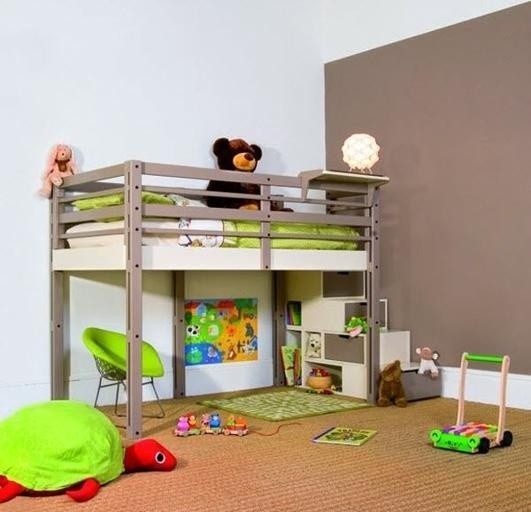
top-left (82, 328), bottom-right (167, 429)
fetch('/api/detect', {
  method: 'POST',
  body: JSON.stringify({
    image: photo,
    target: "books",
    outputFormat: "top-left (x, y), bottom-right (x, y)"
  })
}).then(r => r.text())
top-left (287, 302), bottom-right (301, 325)
top-left (312, 426), bottom-right (378, 447)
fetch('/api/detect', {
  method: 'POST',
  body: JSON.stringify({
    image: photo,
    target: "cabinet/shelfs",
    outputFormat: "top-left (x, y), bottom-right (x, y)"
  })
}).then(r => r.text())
top-left (272, 265), bottom-right (442, 406)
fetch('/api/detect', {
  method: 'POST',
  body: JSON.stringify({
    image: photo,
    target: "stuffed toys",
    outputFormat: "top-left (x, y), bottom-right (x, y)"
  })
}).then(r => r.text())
top-left (307, 332), bottom-right (322, 357)
top-left (344, 315), bottom-right (368, 338)
top-left (1, 398), bottom-right (177, 504)
top-left (416, 345), bottom-right (441, 380)
top-left (37, 143), bottom-right (82, 200)
top-left (377, 360), bottom-right (410, 408)
top-left (201, 135), bottom-right (295, 214)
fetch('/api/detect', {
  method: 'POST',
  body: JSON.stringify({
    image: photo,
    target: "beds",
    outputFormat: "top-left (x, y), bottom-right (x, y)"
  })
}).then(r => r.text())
top-left (50, 158), bottom-right (392, 441)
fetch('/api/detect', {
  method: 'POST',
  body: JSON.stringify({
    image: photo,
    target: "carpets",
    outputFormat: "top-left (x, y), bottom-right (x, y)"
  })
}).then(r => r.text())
top-left (197, 387), bottom-right (380, 423)
